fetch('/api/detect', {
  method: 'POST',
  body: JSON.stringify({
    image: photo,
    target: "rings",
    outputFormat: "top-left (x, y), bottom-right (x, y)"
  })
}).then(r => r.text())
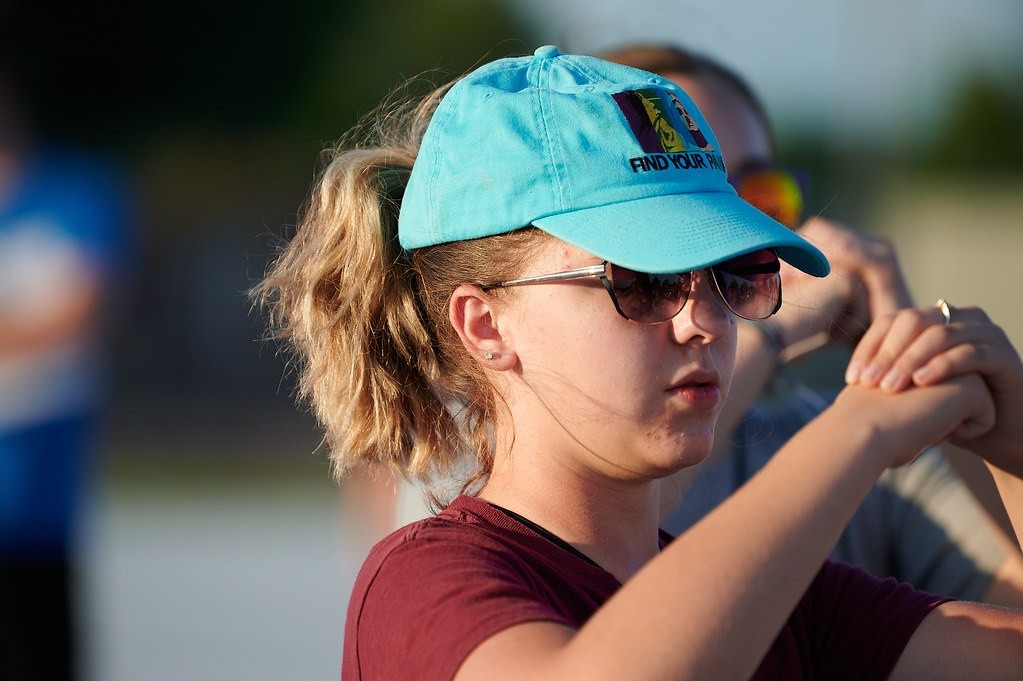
top-left (936, 297), bottom-right (951, 324)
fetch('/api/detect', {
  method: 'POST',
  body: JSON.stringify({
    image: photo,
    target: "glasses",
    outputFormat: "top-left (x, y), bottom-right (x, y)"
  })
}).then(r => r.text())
top-left (479, 260), bottom-right (783, 323)
top-left (724, 160), bottom-right (812, 232)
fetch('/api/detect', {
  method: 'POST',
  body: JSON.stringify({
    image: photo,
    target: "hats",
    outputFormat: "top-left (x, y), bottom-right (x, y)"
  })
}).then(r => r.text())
top-left (396, 44), bottom-right (832, 275)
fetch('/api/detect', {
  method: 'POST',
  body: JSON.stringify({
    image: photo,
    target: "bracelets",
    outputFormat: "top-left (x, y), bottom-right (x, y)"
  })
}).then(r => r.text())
top-left (742, 319), bottom-right (780, 347)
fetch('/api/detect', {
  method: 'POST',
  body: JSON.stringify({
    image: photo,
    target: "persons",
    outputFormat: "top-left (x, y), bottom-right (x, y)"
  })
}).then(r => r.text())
top-left (0, 136), bottom-right (123, 681)
top-left (248, 44), bottom-right (1023, 681)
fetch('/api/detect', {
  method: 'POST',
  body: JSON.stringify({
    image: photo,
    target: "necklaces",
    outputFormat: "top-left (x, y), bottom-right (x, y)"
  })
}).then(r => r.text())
top-left (490, 502), bottom-right (602, 571)
